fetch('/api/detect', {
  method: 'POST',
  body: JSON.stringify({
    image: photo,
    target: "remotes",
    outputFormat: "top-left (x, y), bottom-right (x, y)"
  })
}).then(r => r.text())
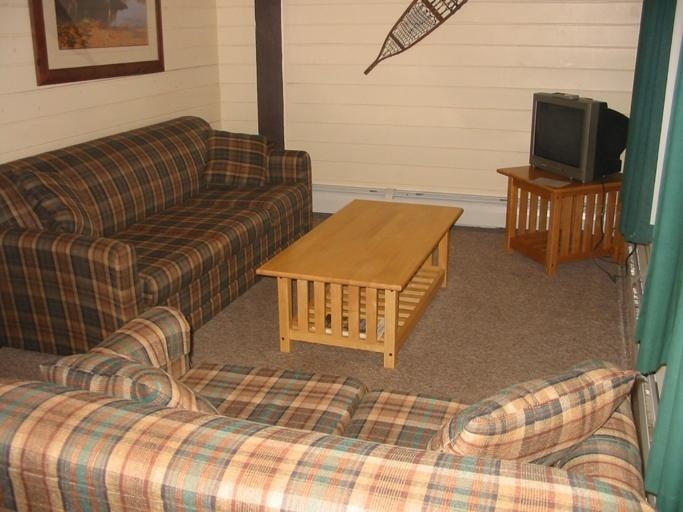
top-left (554, 92), bottom-right (581, 100)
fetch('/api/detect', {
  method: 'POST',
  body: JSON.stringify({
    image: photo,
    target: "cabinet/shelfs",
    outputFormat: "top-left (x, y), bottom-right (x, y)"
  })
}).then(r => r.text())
top-left (497, 166), bottom-right (625, 278)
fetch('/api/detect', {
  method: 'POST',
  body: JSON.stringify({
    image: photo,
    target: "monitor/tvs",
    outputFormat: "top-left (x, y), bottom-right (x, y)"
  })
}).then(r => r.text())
top-left (529, 92), bottom-right (630, 184)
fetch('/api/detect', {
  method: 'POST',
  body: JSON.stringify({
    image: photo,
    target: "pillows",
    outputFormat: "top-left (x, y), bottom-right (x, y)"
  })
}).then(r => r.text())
top-left (39, 355), bottom-right (222, 417)
top-left (13, 169), bottom-right (112, 237)
top-left (427, 359), bottom-right (647, 465)
top-left (204, 130), bottom-right (271, 188)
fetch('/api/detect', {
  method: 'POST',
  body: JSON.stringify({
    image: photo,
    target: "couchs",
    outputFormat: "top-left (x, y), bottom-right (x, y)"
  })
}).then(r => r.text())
top-left (0, 306), bottom-right (654, 512)
top-left (0, 115), bottom-right (312, 356)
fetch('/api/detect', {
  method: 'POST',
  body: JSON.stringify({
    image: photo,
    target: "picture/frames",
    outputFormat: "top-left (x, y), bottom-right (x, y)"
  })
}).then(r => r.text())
top-left (28, 0), bottom-right (165, 86)
